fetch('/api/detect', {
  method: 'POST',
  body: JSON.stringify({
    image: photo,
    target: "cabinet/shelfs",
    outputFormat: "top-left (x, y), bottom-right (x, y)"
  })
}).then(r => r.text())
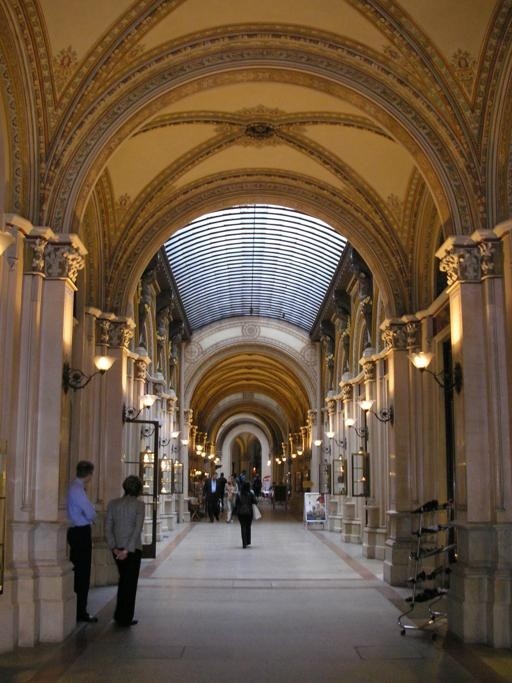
top-left (396, 504), bottom-right (454, 642)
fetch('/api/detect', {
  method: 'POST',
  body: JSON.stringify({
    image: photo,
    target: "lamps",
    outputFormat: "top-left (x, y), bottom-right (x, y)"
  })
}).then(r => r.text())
top-left (355, 398), bottom-right (394, 427)
top-left (122, 392), bottom-right (159, 423)
top-left (405, 351), bottom-right (463, 394)
top-left (63, 354), bottom-right (117, 394)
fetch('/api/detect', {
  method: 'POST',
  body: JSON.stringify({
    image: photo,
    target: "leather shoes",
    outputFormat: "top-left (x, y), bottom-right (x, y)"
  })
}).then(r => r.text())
top-left (76, 613), bottom-right (98, 623)
top-left (115, 618), bottom-right (139, 627)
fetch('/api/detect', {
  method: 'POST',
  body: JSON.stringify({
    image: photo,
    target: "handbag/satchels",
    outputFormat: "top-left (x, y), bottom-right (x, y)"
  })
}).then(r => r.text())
top-left (252, 504), bottom-right (261, 521)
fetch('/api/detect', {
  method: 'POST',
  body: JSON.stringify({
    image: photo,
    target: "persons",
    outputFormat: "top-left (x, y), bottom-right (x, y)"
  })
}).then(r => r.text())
top-left (252, 476), bottom-right (262, 497)
top-left (236, 481), bottom-right (257, 549)
top-left (217, 472), bottom-right (227, 513)
top-left (203, 471), bottom-right (219, 522)
top-left (105, 474), bottom-right (145, 627)
top-left (224, 473), bottom-right (239, 523)
top-left (240, 471), bottom-right (247, 481)
top-left (67, 460), bottom-right (98, 623)
